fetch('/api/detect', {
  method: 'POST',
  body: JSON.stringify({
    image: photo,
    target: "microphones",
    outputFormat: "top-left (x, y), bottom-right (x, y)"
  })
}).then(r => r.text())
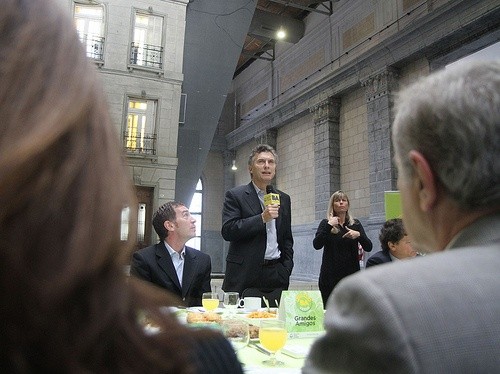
top-left (263, 185), bottom-right (280, 206)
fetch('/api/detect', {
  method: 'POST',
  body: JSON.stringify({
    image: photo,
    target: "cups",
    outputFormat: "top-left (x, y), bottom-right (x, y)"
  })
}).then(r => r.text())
top-left (223, 292), bottom-right (239, 310)
top-left (239, 297), bottom-right (261, 312)
top-left (202, 293), bottom-right (219, 312)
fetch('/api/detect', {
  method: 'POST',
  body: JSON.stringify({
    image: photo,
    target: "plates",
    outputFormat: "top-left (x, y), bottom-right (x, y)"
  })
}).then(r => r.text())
top-left (250, 338), bottom-right (259, 343)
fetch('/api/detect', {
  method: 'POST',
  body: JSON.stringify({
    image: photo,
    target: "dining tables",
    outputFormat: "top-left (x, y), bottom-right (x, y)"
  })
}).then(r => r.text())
top-left (139, 305), bottom-right (329, 374)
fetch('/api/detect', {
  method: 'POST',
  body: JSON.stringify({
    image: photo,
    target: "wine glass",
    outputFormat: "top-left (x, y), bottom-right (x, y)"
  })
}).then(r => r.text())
top-left (222, 320), bottom-right (249, 366)
top-left (259, 320), bottom-right (286, 366)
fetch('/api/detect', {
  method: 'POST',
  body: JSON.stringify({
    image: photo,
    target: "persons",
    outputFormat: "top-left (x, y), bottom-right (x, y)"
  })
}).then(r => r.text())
top-left (130, 201), bottom-right (212, 306)
top-left (221, 143), bottom-right (294, 307)
top-left (0, 0), bottom-right (244, 373)
top-left (300, 60), bottom-right (500, 374)
top-left (366, 218), bottom-right (424, 268)
top-left (313, 190), bottom-right (372, 309)
